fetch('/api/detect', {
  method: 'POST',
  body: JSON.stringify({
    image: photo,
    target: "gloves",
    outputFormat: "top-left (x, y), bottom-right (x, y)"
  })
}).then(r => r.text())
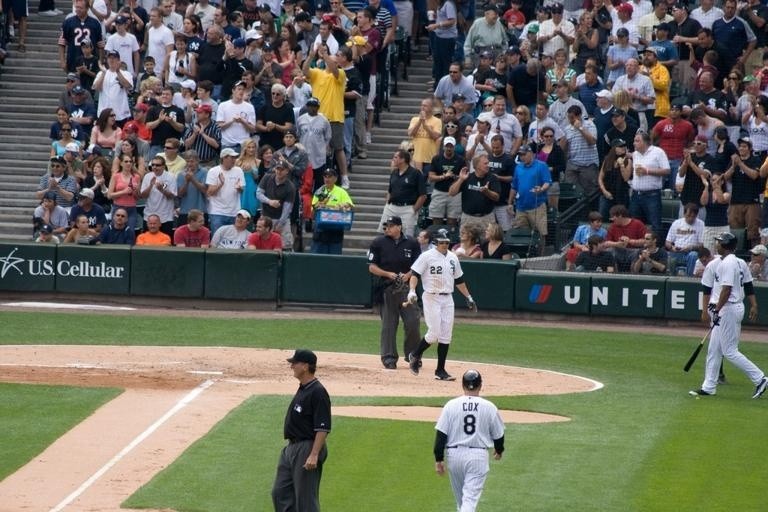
top-left (707, 303), bottom-right (716, 313)
top-left (464, 295), bottom-right (477, 313)
top-left (407, 289), bottom-right (418, 305)
top-left (712, 310), bottom-right (720, 326)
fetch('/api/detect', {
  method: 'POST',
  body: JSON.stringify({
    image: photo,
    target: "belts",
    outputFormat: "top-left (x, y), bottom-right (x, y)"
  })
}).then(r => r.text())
top-left (432, 293), bottom-right (449, 295)
top-left (471, 213), bottom-right (484, 217)
top-left (290, 438), bottom-right (304, 443)
top-left (393, 202), bottom-right (414, 206)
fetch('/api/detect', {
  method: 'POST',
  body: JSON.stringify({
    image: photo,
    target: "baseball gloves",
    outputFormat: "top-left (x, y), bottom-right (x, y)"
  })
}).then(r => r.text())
top-left (390, 275), bottom-right (407, 295)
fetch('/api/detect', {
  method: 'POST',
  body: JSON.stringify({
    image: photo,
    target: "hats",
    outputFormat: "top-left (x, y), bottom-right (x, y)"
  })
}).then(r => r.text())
top-left (39, 15), bottom-right (331, 245)
top-left (749, 245), bottom-right (768, 258)
top-left (286, 348), bottom-right (317, 365)
top-left (382, 216), bottom-right (402, 226)
top-left (440, 2), bottom-right (766, 210)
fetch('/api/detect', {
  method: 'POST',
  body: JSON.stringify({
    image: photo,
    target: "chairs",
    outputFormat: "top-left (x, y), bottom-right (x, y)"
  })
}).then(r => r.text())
top-left (412, 183), bottom-right (585, 254)
top-left (132, 142), bottom-right (342, 252)
top-left (380, 25), bottom-right (412, 111)
top-left (579, 193), bottom-right (764, 280)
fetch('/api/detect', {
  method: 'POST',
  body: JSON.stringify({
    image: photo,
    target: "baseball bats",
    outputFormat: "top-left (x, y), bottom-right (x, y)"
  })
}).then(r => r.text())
top-left (683, 316), bottom-right (722, 372)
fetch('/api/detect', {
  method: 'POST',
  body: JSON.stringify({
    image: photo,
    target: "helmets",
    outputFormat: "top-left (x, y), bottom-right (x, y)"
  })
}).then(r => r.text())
top-left (463, 369), bottom-right (482, 390)
top-left (714, 232), bottom-right (737, 251)
top-left (431, 228), bottom-right (451, 245)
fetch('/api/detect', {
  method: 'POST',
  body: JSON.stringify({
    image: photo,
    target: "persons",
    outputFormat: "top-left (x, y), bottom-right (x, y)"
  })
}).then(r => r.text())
top-left (367, 217), bottom-right (423, 370)
top-left (407, 1), bottom-right (768, 283)
top-left (688, 232), bottom-right (768, 399)
top-left (1, 1), bottom-right (55, 65)
top-left (700, 255), bottom-right (727, 387)
top-left (271, 349), bottom-right (331, 512)
top-left (33, 1), bottom-right (428, 255)
top-left (407, 229), bottom-right (478, 383)
top-left (433, 370), bottom-right (505, 512)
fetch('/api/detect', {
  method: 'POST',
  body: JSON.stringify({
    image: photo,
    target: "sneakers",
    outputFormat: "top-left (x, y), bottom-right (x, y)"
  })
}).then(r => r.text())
top-left (6, 8), bottom-right (64, 54)
top-left (719, 375), bottom-right (727, 385)
top-left (404, 356), bottom-right (422, 366)
top-left (341, 131), bottom-right (371, 190)
top-left (408, 352), bottom-right (419, 376)
top-left (427, 54), bottom-right (435, 92)
top-left (385, 362), bottom-right (396, 369)
top-left (751, 376), bottom-right (768, 399)
top-left (689, 389), bottom-right (716, 397)
top-left (435, 369), bottom-right (456, 381)
